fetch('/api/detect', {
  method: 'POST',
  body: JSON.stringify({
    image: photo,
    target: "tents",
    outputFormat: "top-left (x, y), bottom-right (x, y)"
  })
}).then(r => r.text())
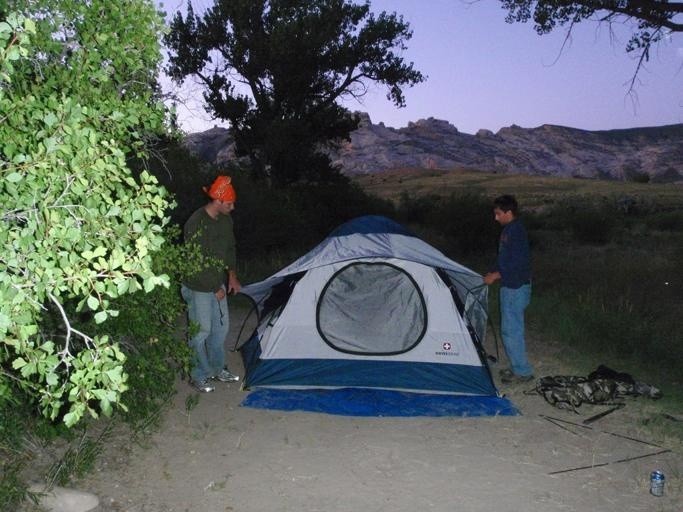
top-left (236, 215), bottom-right (525, 419)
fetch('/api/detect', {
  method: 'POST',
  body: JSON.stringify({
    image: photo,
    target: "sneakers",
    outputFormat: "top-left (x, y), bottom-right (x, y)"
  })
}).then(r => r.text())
top-left (213, 370), bottom-right (239, 382)
top-left (189, 378), bottom-right (215, 393)
top-left (501, 369), bottom-right (515, 378)
top-left (502, 373), bottom-right (533, 385)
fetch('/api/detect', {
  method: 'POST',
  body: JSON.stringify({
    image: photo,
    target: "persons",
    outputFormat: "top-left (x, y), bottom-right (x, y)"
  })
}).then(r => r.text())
top-left (181, 175), bottom-right (241, 395)
top-left (484, 195), bottom-right (536, 387)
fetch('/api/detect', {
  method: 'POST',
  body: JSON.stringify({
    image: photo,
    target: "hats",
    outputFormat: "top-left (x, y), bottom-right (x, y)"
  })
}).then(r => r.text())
top-left (209, 176), bottom-right (236, 201)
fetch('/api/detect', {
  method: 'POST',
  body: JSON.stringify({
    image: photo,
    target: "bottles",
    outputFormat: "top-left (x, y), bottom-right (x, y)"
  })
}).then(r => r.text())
top-left (650, 471), bottom-right (665, 497)
